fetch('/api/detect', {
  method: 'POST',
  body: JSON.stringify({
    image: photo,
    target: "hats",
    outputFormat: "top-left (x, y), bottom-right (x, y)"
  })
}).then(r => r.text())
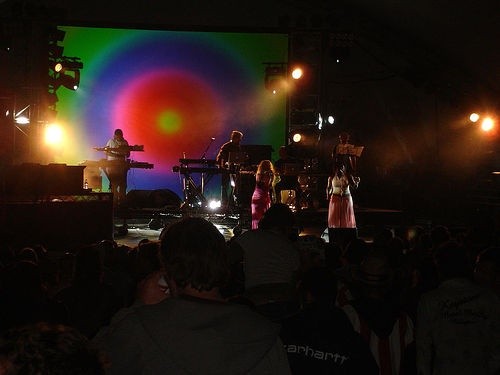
top-left (360, 253), bottom-right (385, 281)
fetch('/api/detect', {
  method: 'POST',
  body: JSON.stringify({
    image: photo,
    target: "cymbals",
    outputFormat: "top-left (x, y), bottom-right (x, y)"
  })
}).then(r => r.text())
top-left (286, 143), bottom-right (320, 158)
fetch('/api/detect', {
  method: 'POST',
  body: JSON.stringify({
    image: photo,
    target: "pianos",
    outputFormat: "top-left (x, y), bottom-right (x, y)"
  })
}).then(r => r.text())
top-left (80, 145), bottom-right (154, 229)
top-left (172, 158), bottom-right (234, 209)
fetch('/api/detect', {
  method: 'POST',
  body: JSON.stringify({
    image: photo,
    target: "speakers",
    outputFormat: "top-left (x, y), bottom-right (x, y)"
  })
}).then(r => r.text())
top-left (236, 145), bottom-right (271, 200)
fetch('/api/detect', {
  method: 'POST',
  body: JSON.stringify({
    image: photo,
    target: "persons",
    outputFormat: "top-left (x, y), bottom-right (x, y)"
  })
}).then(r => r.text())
top-left (107, 129), bottom-right (130, 189)
top-left (250, 160), bottom-right (276, 231)
top-left (215, 130), bottom-right (249, 214)
top-left (324, 160), bottom-right (361, 228)
top-left (0, 203), bottom-right (500, 375)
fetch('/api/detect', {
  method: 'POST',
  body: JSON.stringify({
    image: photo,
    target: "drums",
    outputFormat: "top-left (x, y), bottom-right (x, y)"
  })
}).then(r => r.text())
top-left (300, 199), bottom-right (320, 209)
top-left (300, 175), bottom-right (321, 192)
top-left (228, 151), bottom-right (251, 164)
top-left (281, 161), bottom-right (299, 176)
top-left (238, 170), bottom-right (254, 204)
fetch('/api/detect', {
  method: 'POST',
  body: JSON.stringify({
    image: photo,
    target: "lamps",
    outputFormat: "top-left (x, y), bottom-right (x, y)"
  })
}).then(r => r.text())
top-left (48, 60), bottom-right (63, 74)
top-left (293, 132), bottom-right (307, 145)
